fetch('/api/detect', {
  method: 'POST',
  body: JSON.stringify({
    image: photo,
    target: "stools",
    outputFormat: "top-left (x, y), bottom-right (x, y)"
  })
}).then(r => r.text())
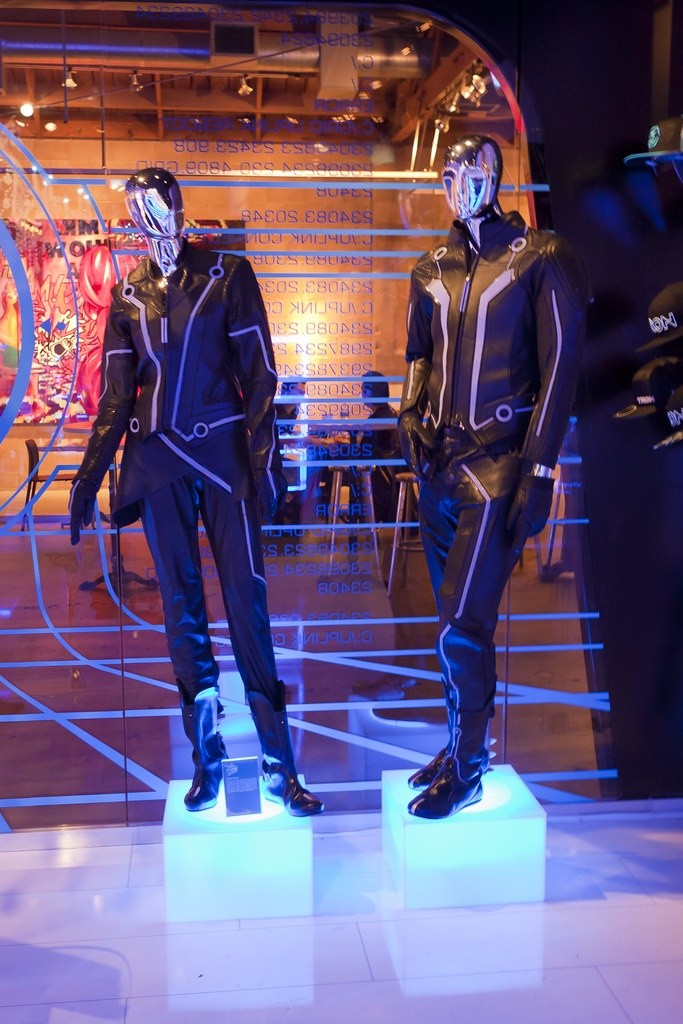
top-left (324, 465), bottom-right (419, 596)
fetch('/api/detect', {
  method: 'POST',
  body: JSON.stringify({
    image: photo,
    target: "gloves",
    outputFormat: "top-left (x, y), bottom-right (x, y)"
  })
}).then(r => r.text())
top-left (398, 411), bottom-right (438, 481)
top-left (506, 473), bottom-right (555, 542)
top-left (253, 469), bottom-right (288, 523)
top-left (68, 478), bottom-right (99, 546)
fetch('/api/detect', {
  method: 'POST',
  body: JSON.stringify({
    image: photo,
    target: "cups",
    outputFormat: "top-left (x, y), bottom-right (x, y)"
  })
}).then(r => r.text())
top-left (322, 414), bottom-right (333, 434)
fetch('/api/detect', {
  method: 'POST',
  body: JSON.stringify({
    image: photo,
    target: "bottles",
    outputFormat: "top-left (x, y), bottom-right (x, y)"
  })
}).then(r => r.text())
top-left (340, 412), bottom-right (350, 435)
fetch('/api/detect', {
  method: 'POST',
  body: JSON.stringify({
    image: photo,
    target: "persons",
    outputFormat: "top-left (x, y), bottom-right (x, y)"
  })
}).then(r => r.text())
top-left (260, 372), bottom-right (414, 538)
top-left (398, 134), bottom-right (584, 819)
top-left (69, 167), bottom-right (324, 818)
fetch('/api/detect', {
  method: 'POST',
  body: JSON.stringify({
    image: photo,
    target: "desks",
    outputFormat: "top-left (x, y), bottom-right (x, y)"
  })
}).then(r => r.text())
top-left (275, 438), bottom-right (364, 519)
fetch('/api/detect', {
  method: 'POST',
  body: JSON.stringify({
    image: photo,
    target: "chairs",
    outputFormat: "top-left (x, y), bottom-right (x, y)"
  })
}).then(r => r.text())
top-left (21, 439), bottom-right (77, 534)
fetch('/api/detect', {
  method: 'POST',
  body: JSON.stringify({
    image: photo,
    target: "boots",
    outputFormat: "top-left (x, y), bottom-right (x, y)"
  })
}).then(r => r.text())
top-left (175, 676), bottom-right (229, 812)
top-left (406, 675), bottom-right (498, 819)
top-left (244, 680), bottom-right (325, 817)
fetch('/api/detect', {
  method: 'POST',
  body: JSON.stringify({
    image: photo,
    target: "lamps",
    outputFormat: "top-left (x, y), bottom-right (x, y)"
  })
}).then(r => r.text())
top-left (61, 71), bottom-right (254, 98)
top-left (461, 71), bottom-right (486, 102)
